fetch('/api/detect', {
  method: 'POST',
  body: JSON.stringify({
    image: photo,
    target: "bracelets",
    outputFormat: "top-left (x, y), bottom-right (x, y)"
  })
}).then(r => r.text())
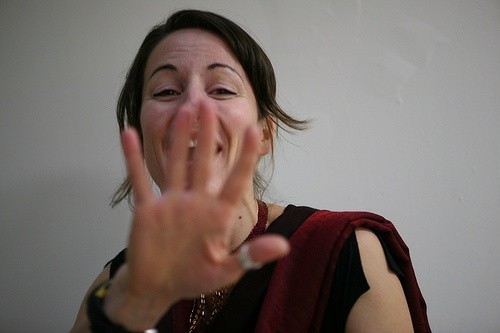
top-left (84, 278), bottom-right (158, 333)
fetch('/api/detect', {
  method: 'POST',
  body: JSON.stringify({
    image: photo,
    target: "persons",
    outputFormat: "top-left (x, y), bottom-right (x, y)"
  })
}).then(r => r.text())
top-left (67, 9), bottom-right (433, 333)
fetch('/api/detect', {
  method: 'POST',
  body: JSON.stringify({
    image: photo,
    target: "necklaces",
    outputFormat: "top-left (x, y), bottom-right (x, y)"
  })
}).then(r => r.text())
top-left (188, 288), bottom-right (230, 333)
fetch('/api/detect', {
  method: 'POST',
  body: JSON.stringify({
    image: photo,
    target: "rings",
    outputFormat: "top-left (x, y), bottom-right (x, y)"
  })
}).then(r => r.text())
top-left (239, 245), bottom-right (264, 271)
